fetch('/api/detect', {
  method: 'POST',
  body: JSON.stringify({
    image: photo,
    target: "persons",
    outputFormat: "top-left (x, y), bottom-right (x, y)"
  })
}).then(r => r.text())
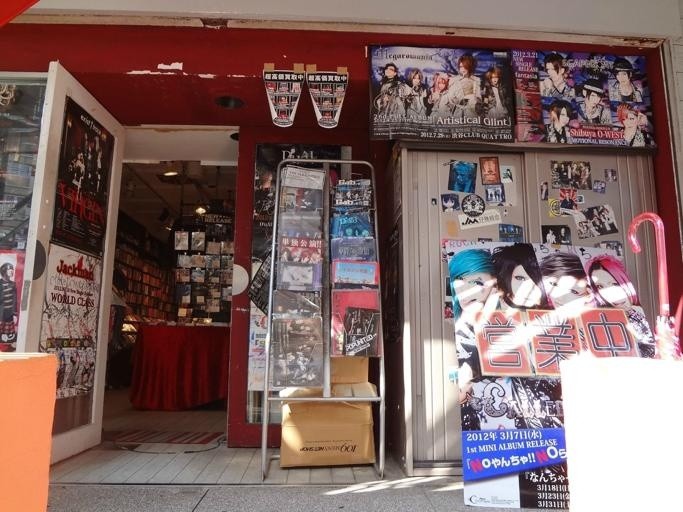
top-left (0, 263), bottom-right (18, 345)
top-left (536, 52), bottom-right (653, 147)
top-left (376, 53), bottom-right (511, 127)
top-left (449, 155), bottom-right (654, 405)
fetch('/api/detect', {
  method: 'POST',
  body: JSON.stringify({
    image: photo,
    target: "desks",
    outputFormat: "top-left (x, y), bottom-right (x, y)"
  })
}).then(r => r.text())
top-left (126, 326), bottom-right (230, 412)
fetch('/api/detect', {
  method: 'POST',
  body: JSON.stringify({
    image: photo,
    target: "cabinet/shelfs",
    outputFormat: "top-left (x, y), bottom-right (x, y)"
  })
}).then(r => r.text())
top-left (106, 209), bottom-right (176, 322)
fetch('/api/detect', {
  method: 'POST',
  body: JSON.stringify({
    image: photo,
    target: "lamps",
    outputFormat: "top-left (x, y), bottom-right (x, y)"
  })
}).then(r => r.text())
top-left (143, 161), bottom-right (219, 215)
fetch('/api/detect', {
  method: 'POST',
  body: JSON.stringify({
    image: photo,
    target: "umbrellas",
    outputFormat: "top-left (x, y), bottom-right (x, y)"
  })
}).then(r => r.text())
top-left (628, 211), bottom-right (682, 359)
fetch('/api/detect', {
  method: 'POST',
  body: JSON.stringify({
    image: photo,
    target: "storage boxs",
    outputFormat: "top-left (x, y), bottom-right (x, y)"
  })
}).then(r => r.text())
top-left (278, 356), bottom-right (382, 468)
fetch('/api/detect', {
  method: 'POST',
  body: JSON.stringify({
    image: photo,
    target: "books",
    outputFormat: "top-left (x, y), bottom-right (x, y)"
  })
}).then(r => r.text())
top-left (113, 229), bottom-right (172, 322)
